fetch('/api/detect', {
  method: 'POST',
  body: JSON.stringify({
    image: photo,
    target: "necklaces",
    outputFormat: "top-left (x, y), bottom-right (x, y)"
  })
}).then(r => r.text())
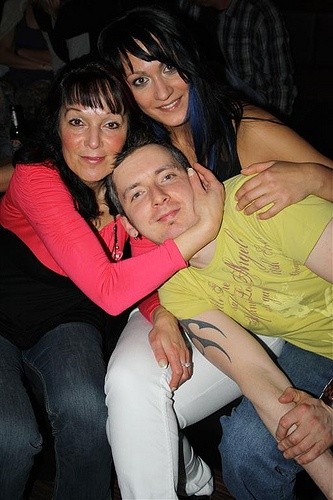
top-left (107, 215), bottom-right (123, 262)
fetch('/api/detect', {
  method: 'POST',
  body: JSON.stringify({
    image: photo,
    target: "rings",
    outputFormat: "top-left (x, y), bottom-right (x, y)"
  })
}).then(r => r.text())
top-left (181, 362), bottom-right (193, 368)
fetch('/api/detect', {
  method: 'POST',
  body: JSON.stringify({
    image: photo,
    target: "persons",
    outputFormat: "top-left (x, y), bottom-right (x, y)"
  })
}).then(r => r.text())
top-left (1, 0), bottom-right (130, 160)
top-left (0, 54), bottom-right (225, 499)
top-left (99, 132), bottom-right (333, 500)
top-left (0, 7), bottom-right (333, 500)
top-left (170, 0), bottom-right (298, 123)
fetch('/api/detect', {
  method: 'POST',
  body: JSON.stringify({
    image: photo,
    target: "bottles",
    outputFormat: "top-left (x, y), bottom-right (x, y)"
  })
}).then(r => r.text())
top-left (10, 104), bottom-right (25, 152)
